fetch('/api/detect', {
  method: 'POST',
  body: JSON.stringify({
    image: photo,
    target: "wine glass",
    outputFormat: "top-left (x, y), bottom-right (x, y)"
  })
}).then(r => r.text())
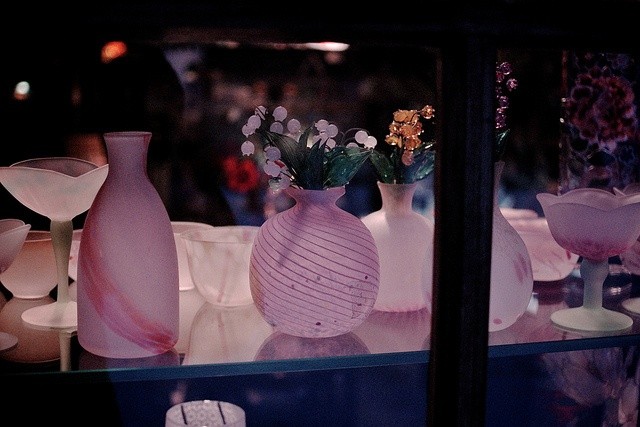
top-left (1, 219), bottom-right (30, 352)
top-left (1, 157), bottom-right (110, 330)
top-left (619, 240), bottom-right (639, 314)
top-left (536, 188), bottom-right (640, 336)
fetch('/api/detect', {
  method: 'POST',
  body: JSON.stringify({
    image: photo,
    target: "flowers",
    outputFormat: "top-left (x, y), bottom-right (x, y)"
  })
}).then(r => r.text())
top-left (495, 61), bottom-right (518, 161)
top-left (366, 105), bottom-right (435, 183)
top-left (240, 105), bottom-right (377, 190)
top-left (560, 48), bottom-right (638, 153)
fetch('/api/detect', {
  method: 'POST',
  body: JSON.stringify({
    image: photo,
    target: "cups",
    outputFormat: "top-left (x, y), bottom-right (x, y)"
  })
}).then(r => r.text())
top-left (184, 225), bottom-right (260, 308)
top-left (500, 208), bottom-right (536, 221)
top-left (169, 221), bottom-right (212, 292)
top-left (507, 219), bottom-right (580, 282)
top-left (1, 232), bottom-right (58, 299)
top-left (66, 229), bottom-right (83, 285)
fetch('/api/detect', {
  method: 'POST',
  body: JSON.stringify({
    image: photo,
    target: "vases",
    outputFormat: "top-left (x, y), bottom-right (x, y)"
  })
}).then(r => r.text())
top-left (248, 186), bottom-right (380, 339)
top-left (421, 159), bottom-right (534, 332)
top-left (359, 181), bottom-right (433, 312)
